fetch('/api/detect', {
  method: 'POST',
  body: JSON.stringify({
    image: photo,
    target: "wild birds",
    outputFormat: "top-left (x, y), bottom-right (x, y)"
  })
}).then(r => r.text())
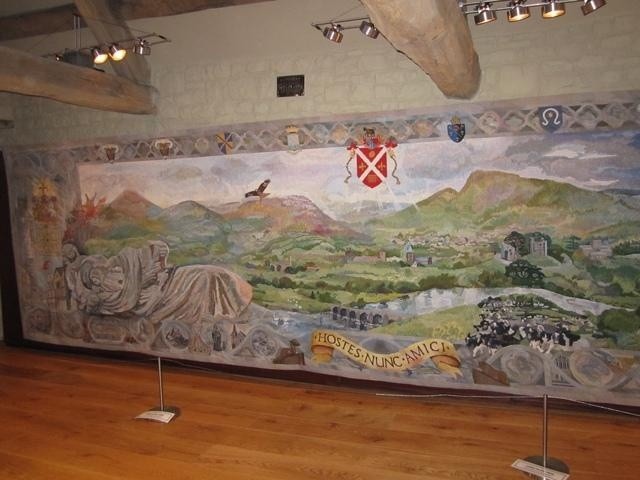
top-left (245, 179), bottom-right (271, 205)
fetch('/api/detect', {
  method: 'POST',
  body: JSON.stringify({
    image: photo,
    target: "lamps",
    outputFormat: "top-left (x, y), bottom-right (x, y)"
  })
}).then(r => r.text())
top-left (25, 10), bottom-right (172, 66)
top-left (310, 1), bottom-right (609, 43)
